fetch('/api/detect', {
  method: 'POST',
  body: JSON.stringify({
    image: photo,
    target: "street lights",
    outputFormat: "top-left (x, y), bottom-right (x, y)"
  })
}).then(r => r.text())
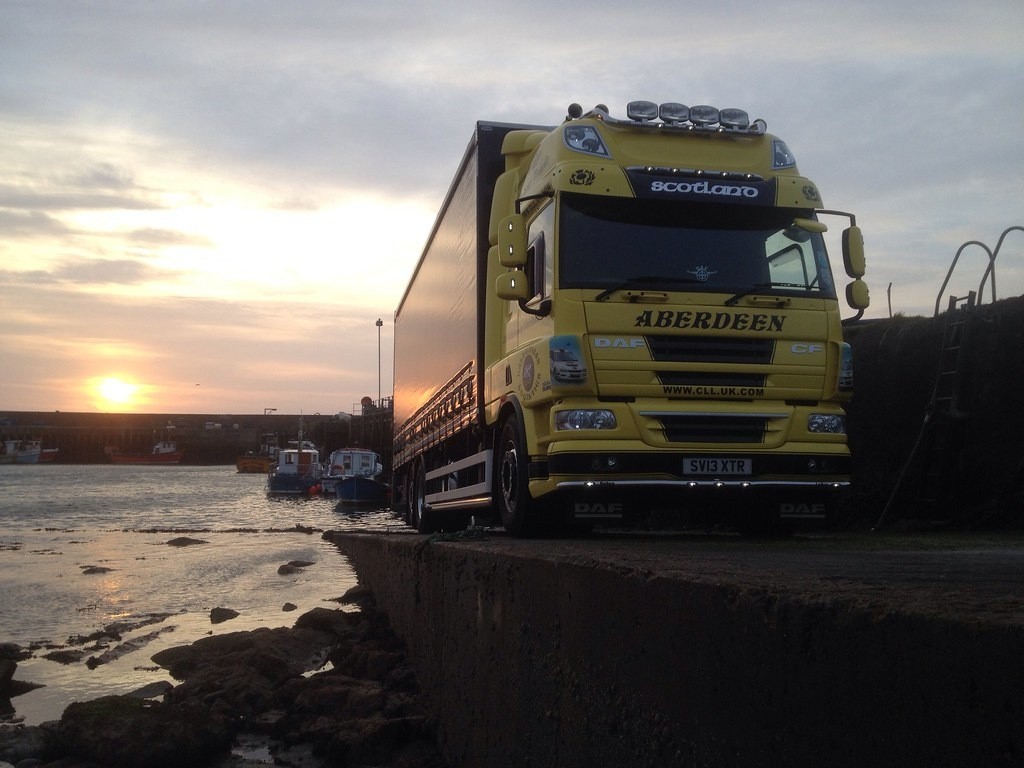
top-left (375, 318), bottom-right (383, 407)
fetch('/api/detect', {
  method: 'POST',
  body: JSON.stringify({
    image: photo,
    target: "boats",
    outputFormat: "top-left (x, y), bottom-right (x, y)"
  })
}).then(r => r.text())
top-left (266, 440), bottom-right (325, 497)
top-left (322, 446), bottom-right (384, 494)
top-left (235, 453), bottom-right (270, 474)
top-left (109, 440), bottom-right (183, 465)
top-left (0, 439), bottom-right (59, 464)
top-left (333, 475), bottom-right (378, 505)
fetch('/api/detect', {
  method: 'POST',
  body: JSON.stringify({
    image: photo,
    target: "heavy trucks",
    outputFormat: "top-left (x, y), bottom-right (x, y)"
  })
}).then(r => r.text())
top-left (389, 100), bottom-right (871, 536)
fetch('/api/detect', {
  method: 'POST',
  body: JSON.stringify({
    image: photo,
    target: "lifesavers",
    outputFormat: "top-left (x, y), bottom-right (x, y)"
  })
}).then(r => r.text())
top-left (331, 464), bottom-right (344, 475)
